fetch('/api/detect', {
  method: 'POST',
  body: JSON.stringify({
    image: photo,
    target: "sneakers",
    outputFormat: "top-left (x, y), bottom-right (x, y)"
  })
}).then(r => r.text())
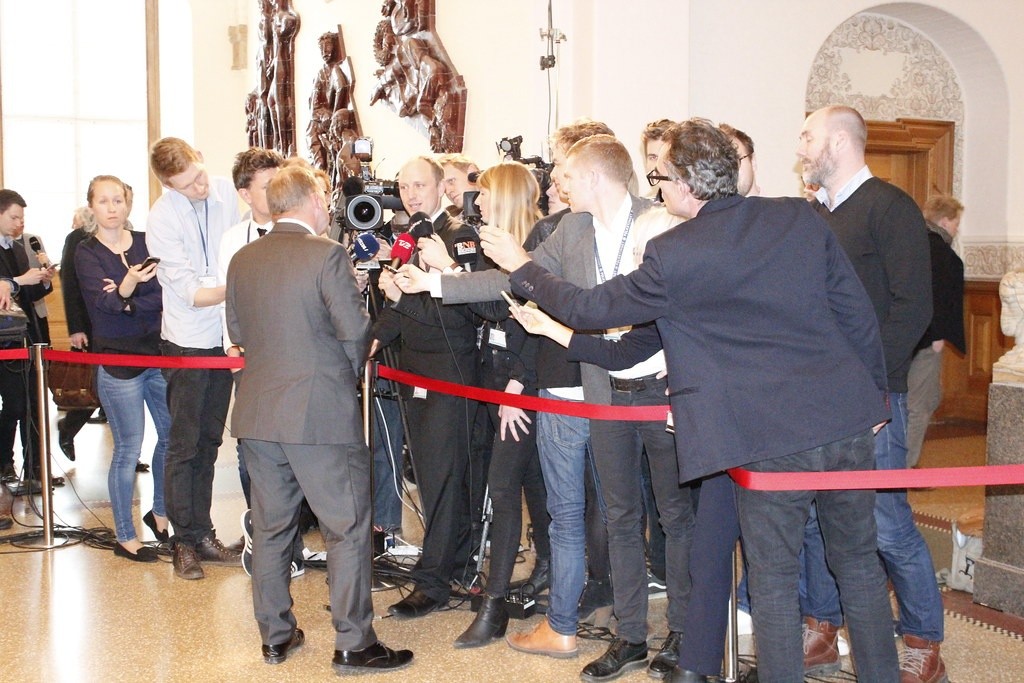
top-left (195, 536), bottom-right (242, 566)
top-left (173, 540), bottom-right (204, 579)
top-left (242, 509), bottom-right (253, 575)
top-left (291, 557), bottom-right (305, 577)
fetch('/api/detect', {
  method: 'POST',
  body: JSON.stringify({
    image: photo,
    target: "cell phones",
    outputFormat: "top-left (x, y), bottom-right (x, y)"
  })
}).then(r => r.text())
top-left (46, 264), bottom-right (60, 271)
top-left (139, 257), bottom-right (161, 272)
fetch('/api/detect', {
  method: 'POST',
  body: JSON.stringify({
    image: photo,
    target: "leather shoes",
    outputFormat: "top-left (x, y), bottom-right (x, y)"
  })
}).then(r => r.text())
top-left (647, 631), bottom-right (683, 677)
top-left (506, 617), bottom-right (578, 658)
top-left (901, 635), bottom-right (947, 683)
top-left (136, 460), bottom-right (150, 471)
top-left (0, 466), bottom-right (20, 486)
top-left (388, 588), bottom-right (448, 618)
top-left (58, 419), bottom-right (75, 461)
top-left (581, 639), bottom-right (648, 682)
top-left (799, 615), bottom-right (842, 677)
top-left (262, 628), bottom-right (305, 664)
top-left (24, 468), bottom-right (65, 485)
top-left (333, 641), bottom-right (413, 676)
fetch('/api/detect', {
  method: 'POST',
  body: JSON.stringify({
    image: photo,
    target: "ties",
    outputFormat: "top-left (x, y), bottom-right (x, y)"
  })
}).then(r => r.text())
top-left (257, 228), bottom-right (267, 238)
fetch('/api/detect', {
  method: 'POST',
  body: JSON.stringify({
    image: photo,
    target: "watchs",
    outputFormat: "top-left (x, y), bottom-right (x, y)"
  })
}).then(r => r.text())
top-left (443, 262), bottom-right (460, 274)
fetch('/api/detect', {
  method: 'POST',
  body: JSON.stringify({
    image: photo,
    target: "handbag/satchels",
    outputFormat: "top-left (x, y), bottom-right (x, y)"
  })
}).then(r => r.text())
top-left (50, 357), bottom-right (99, 411)
top-left (936, 524), bottom-right (982, 593)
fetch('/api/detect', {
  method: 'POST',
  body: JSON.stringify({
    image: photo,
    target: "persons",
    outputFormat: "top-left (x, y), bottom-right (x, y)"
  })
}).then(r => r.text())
top-left (225, 165), bottom-right (414, 671)
top-left (0, 189), bottom-right (65, 486)
top-left (217, 147), bottom-right (306, 577)
top-left (71, 206), bottom-right (107, 423)
top-left (316, 121), bottom-right (769, 683)
top-left (907, 195), bottom-right (967, 490)
top-left (478, 117), bottom-right (899, 683)
top-left (74, 174), bottom-right (170, 561)
top-left (306, 32), bottom-right (346, 169)
top-left (795, 105), bottom-right (951, 683)
top-left (256, 0), bottom-right (295, 155)
top-left (57, 182), bottom-right (150, 470)
top-left (145, 137), bottom-right (243, 579)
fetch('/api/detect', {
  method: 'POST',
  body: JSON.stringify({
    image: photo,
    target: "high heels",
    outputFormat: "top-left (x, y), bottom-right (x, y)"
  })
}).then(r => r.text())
top-left (577, 578), bottom-right (614, 627)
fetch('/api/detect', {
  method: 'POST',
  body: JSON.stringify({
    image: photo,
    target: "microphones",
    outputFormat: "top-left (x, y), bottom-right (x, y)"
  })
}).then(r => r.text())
top-left (342, 176), bottom-right (364, 196)
top-left (385, 234), bottom-right (415, 299)
top-left (349, 235), bottom-right (380, 264)
top-left (409, 212), bottom-right (434, 241)
top-left (29, 237), bottom-right (46, 268)
top-left (453, 237), bottom-right (476, 273)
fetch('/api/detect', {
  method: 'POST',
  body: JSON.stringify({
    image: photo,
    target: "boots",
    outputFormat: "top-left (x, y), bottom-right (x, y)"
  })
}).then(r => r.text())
top-left (521, 558), bottom-right (550, 595)
top-left (452, 595), bottom-right (509, 648)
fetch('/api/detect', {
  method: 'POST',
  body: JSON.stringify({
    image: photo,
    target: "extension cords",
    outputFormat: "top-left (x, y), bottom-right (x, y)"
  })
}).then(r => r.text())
top-left (387, 546), bottom-right (420, 555)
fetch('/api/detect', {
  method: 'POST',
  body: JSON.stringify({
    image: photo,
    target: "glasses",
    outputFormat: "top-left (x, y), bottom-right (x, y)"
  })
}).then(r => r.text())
top-left (647, 170), bottom-right (680, 186)
top-left (736, 152), bottom-right (752, 168)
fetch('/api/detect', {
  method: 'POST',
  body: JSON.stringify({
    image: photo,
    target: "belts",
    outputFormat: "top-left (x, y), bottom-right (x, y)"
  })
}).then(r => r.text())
top-left (609, 376), bottom-right (647, 392)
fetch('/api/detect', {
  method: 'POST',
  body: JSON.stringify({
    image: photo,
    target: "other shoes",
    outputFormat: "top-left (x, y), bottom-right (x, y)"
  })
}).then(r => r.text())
top-left (114, 542), bottom-right (160, 562)
top-left (648, 571), bottom-right (668, 600)
top-left (144, 510), bottom-right (168, 543)
top-left (0, 516), bottom-right (13, 530)
